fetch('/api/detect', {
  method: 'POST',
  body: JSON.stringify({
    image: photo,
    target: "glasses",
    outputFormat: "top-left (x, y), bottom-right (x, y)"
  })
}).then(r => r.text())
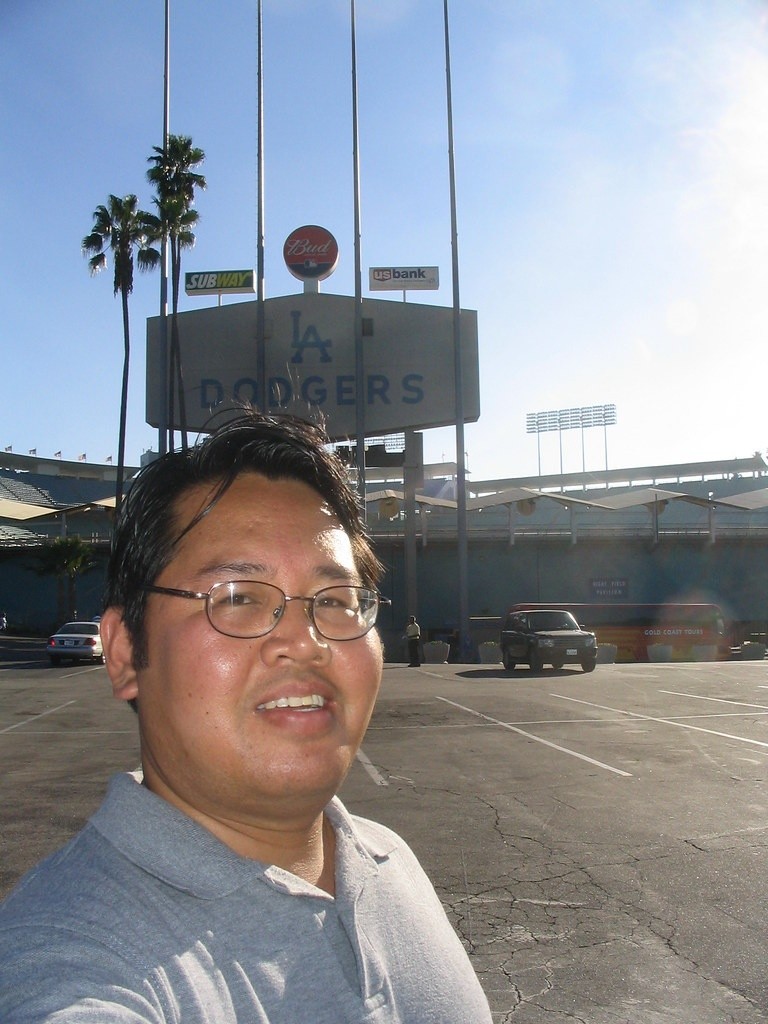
top-left (123, 579), bottom-right (392, 644)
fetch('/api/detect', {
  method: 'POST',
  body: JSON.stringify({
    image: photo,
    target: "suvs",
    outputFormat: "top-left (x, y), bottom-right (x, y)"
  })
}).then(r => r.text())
top-left (499, 610), bottom-right (598, 672)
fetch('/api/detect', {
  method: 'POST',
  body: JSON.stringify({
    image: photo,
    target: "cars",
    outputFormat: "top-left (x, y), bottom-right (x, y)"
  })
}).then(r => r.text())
top-left (46, 621), bottom-right (106, 666)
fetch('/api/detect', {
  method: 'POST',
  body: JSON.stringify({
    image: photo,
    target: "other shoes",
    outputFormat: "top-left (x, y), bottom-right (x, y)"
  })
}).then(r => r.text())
top-left (408, 664), bottom-right (420, 667)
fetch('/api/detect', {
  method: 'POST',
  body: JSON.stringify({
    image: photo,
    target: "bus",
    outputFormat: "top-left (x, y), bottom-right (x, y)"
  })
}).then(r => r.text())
top-left (511, 602), bottom-right (732, 663)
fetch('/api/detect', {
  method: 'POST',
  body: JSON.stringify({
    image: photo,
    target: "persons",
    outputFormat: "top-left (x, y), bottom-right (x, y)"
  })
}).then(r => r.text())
top-left (0, 403), bottom-right (493, 1023)
top-left (2, 615), bottom-right (8, 634)
top-left (401, 615), bottom-right (422, 667)
